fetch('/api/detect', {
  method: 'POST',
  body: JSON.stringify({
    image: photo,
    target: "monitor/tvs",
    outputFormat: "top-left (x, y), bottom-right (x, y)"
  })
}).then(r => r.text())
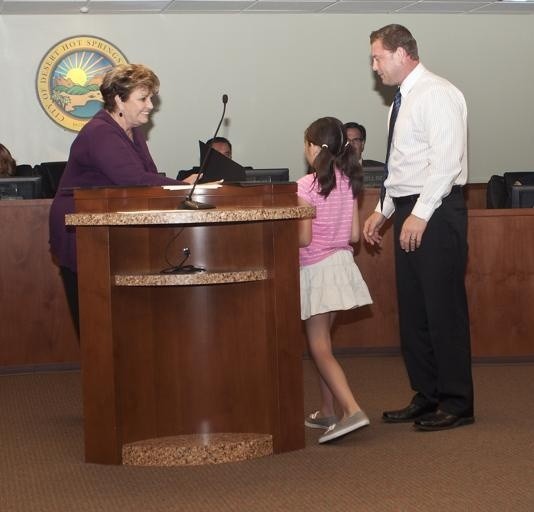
top-left (0, 177), bottom-right (42, 199)
top-left (512, 186), bottom-right (534, 208)
top-left (245, 169), bottom-right (289, 181)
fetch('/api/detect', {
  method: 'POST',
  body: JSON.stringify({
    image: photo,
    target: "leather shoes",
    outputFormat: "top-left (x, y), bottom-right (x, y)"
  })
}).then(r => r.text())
top-left (415, 410), bottom-right (474, 429)
top-left (382, 401), bottom-right (428, 422)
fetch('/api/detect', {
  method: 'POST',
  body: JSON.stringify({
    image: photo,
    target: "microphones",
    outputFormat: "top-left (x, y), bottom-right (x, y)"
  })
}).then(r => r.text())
top-left (183, 94), bottom-right (229, 209)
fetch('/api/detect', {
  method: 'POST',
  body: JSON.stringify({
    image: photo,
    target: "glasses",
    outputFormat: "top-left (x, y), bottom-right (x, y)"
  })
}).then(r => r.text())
top-left (346, 137), bottom-right (363, 144)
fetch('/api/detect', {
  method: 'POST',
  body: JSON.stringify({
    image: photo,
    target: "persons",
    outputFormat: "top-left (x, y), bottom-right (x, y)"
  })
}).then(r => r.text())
top-left (49, 63), bottom-right (225, 345)
top-left (0, 143), bottom-right (16, 178)
top-left (206, 137), bottom-right (232, 158)
top-left (362, 23), bottom-right (476, 430)
top-left (342, 122), bottom-right (385, 166)
top-left (297, 117), bottom-right (373, 445)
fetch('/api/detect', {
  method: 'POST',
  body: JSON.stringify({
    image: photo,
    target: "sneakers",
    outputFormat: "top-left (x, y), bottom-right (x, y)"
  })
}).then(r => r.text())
top-left (319, 411), bottom-right (369, 444)
top-left (305, 411), bottom-right (339, 430)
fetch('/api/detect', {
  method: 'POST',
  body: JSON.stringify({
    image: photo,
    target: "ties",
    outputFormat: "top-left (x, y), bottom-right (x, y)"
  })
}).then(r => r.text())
top-left (381, 88), bottom-right (401, 210)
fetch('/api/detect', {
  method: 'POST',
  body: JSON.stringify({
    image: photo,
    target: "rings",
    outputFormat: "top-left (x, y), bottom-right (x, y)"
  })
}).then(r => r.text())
top-left (411, 239), bottom-right (416, 241)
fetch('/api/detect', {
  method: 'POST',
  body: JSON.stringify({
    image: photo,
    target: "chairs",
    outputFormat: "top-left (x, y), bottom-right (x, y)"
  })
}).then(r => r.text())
top-left (504, 171), bottom-right (533, 208)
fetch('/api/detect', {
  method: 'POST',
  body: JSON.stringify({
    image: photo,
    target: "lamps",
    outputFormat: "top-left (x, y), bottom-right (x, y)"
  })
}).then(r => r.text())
top-left (81, 7), bottom-right (89, 13)
